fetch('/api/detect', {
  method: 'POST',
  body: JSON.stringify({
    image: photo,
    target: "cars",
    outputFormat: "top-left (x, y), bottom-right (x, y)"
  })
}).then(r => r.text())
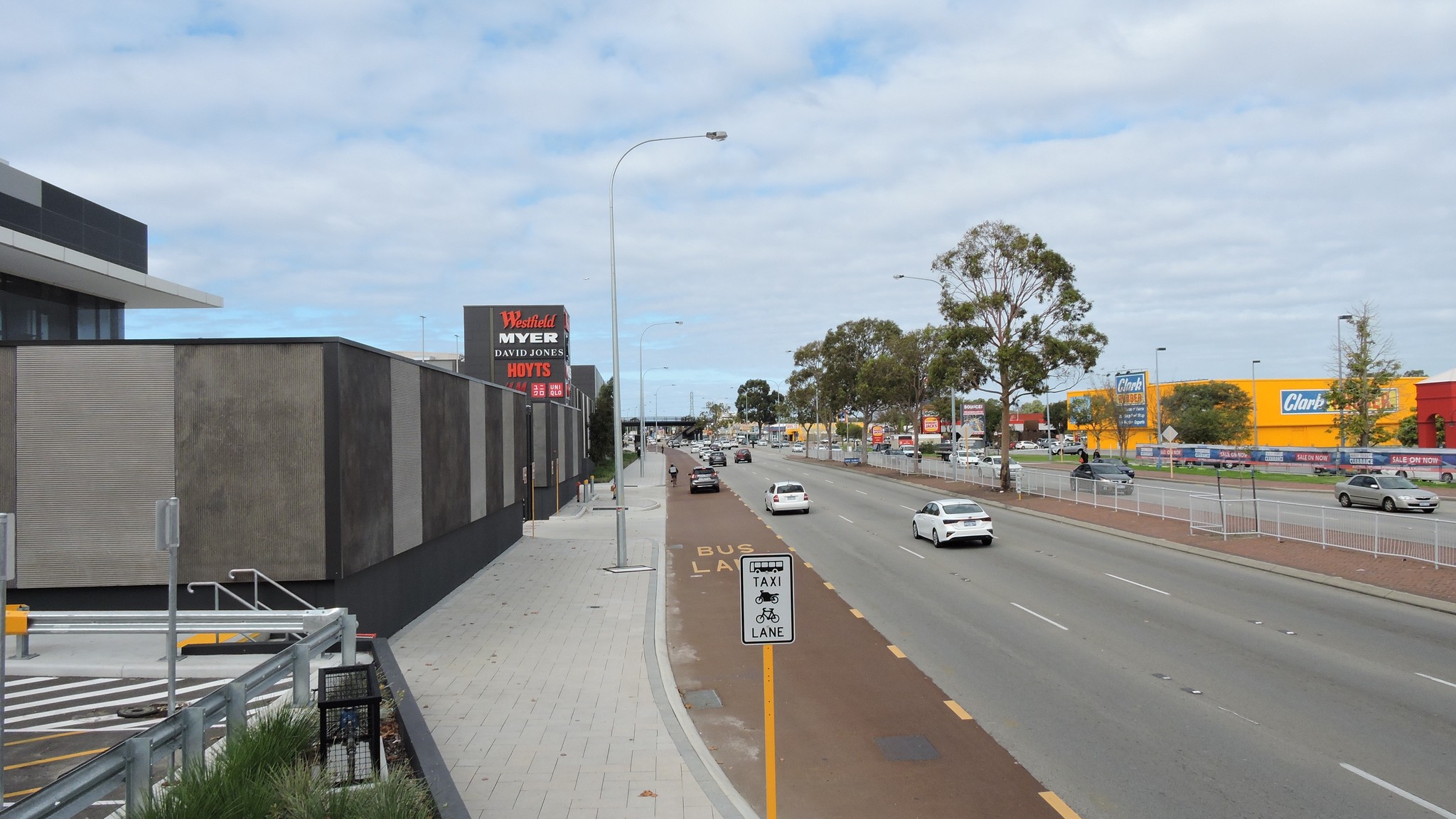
top-left (1171, 448), bottom-right (1252, 468)
top-left (1370, 459), bottom-right (1456, 483)
top-left (912, 499), bottom-right (994, 549)
top-left (764, 481), bottom-right (810, 516)
top-left (1069, 462), bottom-right (1134, 495)
top-left (1091, 458), bottom-right (1135, 478)
top-left (623, 432), bottom-right (922, 463)
top-left (1312, 453), bottom-right (1391, 476)
top-left (1333, 473), bottom-right (1440, 514)
top-left (687, 465), bottom-right (720, 494)
top-left (934, 434), bottom-right (1087, 481)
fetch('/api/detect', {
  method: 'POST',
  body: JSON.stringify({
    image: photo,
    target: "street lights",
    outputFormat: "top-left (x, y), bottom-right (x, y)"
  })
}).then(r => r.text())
top-left (785, 350), bottom-right (820, 459)
top-left (1156, 347), bottom-right (1167, 465)
top-left (610, 130), bottom-right (730, 567)
top-left (1337, 314), bottom-right (1354, 477)
top-left (893, 274), bottom-right (1009, 481)
top-left (759, 379), bottom-right (790, 453)
top-left (455, 335), bottom-right (459, 373)
top-left (1252, 360), bottom-right (1261, 461)
top-left (420, 316), bottom-right (426, 362)
top-left (730, 386), bottom-right (763, 448)
top-left (702, 397), bottom-right (728, 440)
top-left (622, 321), bottom-right (683, 478)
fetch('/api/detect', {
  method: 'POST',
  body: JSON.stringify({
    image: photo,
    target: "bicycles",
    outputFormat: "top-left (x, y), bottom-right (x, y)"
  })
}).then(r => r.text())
top-left (670, 473), bottom-right (678, 487)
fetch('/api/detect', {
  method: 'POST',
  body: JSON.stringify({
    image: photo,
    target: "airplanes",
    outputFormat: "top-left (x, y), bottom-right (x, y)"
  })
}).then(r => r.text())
top-left (583, 277), bottom-right (589, 280)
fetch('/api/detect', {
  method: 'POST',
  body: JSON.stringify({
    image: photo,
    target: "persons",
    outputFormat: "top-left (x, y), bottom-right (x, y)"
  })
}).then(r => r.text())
top-left (661, 444), bottom-right (664, 454)
top-left (1080, 447), bottom-right (1088, 464)
top-left (751, 439), bottom-right (754, 448)
top-left (1093, 449), bottom-right (1101, 459)
top-left (637, 449), bottom-right (640, 460)
top-left (625, 441), bottom-right (627, 448)
top-left (623, 441), bottom-right (625, 448)
top-left (669, 463), bottom-right (679, 485)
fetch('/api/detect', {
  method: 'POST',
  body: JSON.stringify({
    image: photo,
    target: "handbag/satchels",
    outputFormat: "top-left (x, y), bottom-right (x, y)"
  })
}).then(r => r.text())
top-left (1079, 458), bottom-right (1084, 463)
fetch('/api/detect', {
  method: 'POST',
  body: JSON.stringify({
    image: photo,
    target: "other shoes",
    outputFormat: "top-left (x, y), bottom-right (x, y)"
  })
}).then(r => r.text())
top-left (671, 480), bottom-right (673, 482)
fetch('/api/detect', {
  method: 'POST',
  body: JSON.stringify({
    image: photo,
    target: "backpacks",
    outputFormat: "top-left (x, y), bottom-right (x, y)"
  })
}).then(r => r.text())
top-left (670, 466), bottom-right (676, 473)
